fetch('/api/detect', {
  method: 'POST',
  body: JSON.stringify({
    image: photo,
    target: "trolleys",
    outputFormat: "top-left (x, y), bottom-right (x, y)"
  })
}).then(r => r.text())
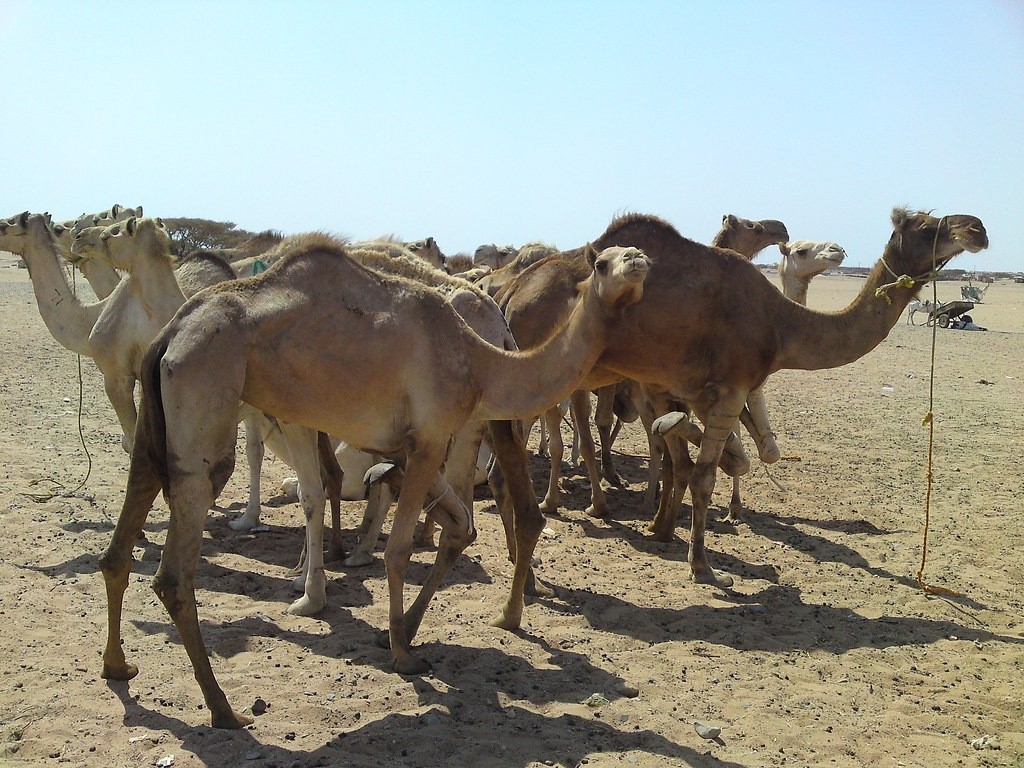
top-left (919, 301), bottom-right (975, 329)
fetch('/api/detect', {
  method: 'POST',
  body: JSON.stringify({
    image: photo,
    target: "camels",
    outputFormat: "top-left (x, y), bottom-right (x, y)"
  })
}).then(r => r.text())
top-left (0, 202), bottom-right (990, 731)
